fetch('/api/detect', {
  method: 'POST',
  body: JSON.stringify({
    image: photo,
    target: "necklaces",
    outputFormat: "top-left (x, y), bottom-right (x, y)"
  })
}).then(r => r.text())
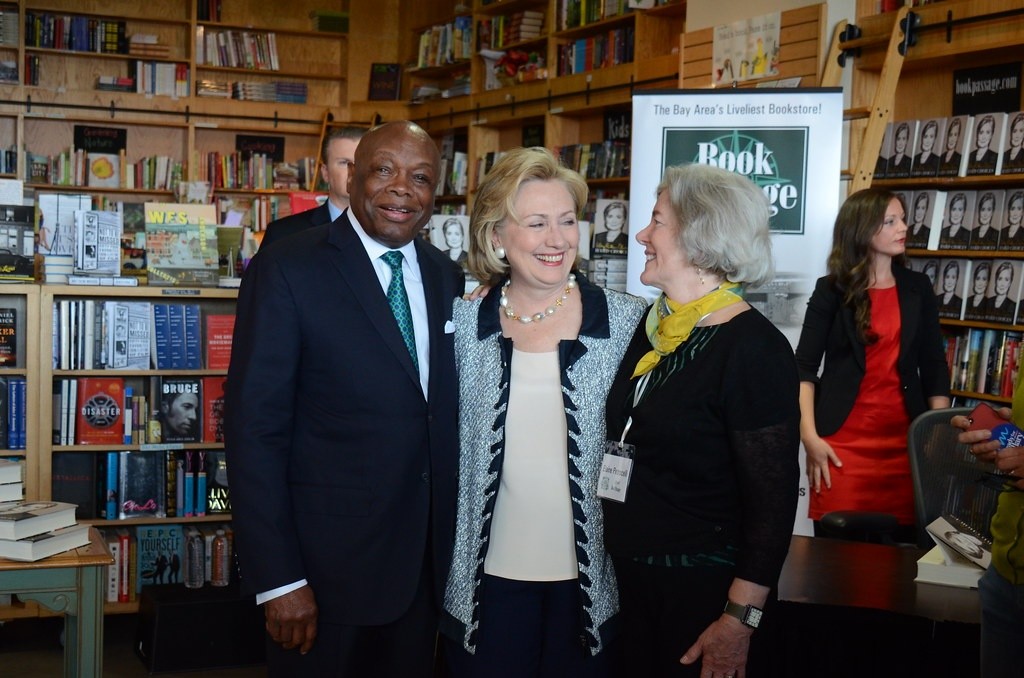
top-left (500, 273), bottom-right (577, 323)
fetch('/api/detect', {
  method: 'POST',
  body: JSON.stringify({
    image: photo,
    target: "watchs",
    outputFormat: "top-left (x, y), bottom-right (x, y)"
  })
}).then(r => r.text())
top-left (723, 599), bottom-right (765, 630)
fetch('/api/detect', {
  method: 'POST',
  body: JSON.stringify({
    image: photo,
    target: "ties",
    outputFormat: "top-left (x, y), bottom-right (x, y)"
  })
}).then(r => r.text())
top-left (381, 250), bottom-right (419, 378)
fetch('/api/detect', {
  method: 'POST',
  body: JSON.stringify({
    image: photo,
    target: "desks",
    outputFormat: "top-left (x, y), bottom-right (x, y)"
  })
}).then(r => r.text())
top-left (772, 534), bottom-right (981, 678)
top-left (0, 528), bottom-right (115, 678)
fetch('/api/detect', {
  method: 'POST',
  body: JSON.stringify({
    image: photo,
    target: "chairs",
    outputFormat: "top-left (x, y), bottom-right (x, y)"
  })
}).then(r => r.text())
top-left (909, 406), bottom-right (1004, 547)
top-left (0, 0), bottom-right (1024, 619)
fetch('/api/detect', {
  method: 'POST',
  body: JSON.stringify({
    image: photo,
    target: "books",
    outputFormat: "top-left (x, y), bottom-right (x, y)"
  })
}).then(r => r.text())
top-left (0, 144), bottom-right (329, 603)
top-left (867, 111), bottom-right (1024, 541)
top-left (430, 205), bottom-right (480, 294)
top-left (914, 511), bottom-right (992, 589)
top-left (0, 0), bottom-right (309, 105)
top-left (410, 0), bottom-right (657, 198)
top-left (576, 199), bottom-right (630, 292)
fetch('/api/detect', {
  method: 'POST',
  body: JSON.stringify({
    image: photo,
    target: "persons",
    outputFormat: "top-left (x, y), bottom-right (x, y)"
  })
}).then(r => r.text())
top-left (225, 120), bottom-right (465, 678)
top-left (603, 164), bottom-right (802, 678)
top-left (794, 189), bottom-right (952, 542)
top-left (950, 340), bottom-right (1024, 677)
top-left (443, 148), bottom-right (648, 677)
